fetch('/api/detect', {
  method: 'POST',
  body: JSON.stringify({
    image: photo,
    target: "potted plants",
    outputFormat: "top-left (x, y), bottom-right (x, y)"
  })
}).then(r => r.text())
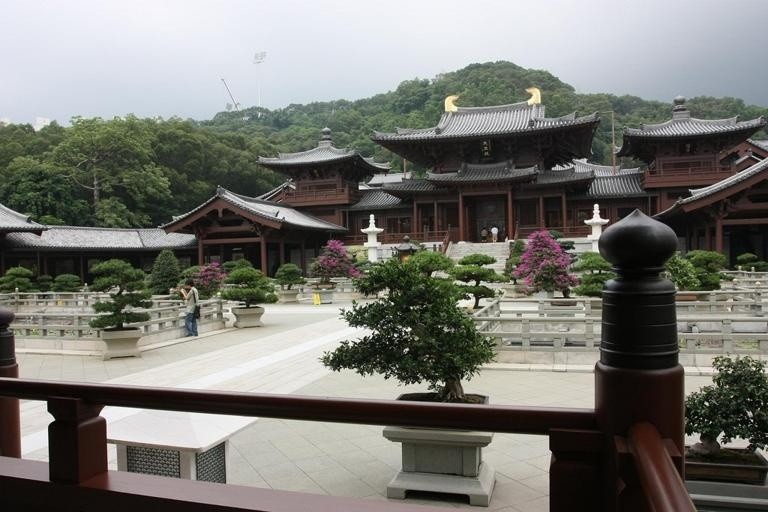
top-left (318, 257), bottom-right (498, 506)
top-left (663, 251), bottom-right (702, 301)
top-left (685, 355), bottom-right (768, 485)
top-left (89, 259), bottom-right (154, 361)
top-left (514, 231), bottom-right (578, 315)
top-left (686, 250), bottom-right (729, 301)
top-left (275, 263), bottom-right (306, 302)
top-left (500, 241), bottom-right (527, 298)
top-left (221, 267), bottom-right (279, 328)
top-left (454, 254), bottom-right (500, 317)
top-left (312, 239), bottom-right (362, 304)
top-left (568, 256), bottom-right (616, 309)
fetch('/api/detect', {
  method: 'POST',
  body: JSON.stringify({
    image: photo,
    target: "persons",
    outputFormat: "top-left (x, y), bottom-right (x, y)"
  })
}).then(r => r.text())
top-left (490, 224), bottom-right (499, 242)
top-left (180, 279), bottom-right (198, 337)
top-left (480, 228), bottom-right (488, 243)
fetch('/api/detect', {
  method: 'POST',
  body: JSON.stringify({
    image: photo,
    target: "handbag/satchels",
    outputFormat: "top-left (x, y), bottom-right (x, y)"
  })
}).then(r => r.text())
top-left (194, 305), bottom-right (201, 318)
